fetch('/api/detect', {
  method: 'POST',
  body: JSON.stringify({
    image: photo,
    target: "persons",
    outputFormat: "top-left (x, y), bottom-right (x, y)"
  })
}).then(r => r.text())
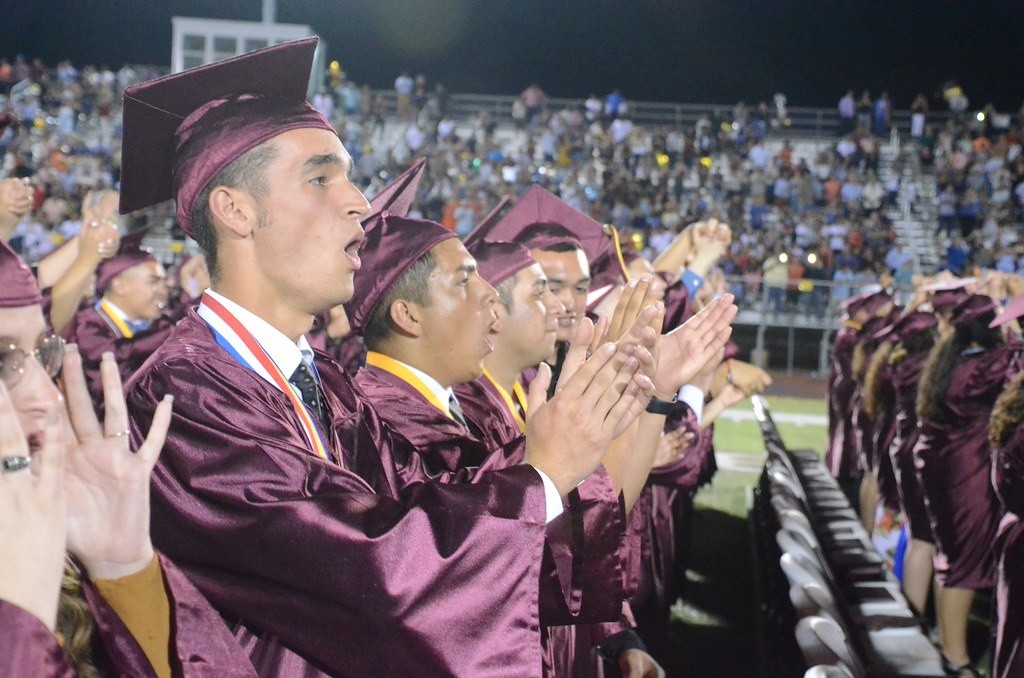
top-left (0, 50), bottom-right (1024, 331)
top-left (95, 32), bottom-right (776, 677)
top-left (0, 242), bottom-right (259, 678)
top-left (824, 268), bottom-right (1024, 678)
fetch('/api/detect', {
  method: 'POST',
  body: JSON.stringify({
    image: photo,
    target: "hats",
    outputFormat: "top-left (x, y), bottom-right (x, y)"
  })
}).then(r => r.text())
top-left (343, 156), bottom-right (459, 335)
top-left (119, 38), bottom-right (338, 238)
top-left (96, 229), bottom-right (156, 294)
top-left (0, 242), bottom-right (42, 308)
top-left (461, 184), bottom-right (640, 317)
top-left (844, 283), bottom-right (1024, 343)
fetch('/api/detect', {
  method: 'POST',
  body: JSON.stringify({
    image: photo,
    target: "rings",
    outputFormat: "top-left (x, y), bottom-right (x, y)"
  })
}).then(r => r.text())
top-left (105, 429), bottom-right (131, 437)
top-left (2, 456), bottom-right (31, 469)
top-left (88, 220), bottom-right (101, 226)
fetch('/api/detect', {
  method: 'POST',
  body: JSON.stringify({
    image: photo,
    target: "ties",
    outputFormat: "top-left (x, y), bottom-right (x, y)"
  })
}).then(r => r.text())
top-left (290, 364), bottom-right (329, 441)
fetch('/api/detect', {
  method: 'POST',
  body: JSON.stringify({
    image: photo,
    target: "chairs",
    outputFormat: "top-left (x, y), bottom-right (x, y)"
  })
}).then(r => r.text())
top-left (878, 146), bottom-right (939, 271)
top-left (752, 393), bottom-right (945, 678)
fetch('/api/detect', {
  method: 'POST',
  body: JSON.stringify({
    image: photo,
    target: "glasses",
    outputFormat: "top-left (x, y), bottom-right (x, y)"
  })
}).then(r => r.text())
top-left (0, 336), bottom-right (64, 391)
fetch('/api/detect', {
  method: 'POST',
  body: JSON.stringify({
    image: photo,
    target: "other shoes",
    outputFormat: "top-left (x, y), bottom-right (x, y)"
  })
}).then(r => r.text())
top-left (929, 626), bottom-right (941, 646)
top-left (941, 647), bottom-right (985, 677)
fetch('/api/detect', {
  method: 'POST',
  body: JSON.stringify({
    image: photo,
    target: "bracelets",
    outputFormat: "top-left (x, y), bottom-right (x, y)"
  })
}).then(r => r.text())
top-left (645, 393), bottom-right (677, 416)
